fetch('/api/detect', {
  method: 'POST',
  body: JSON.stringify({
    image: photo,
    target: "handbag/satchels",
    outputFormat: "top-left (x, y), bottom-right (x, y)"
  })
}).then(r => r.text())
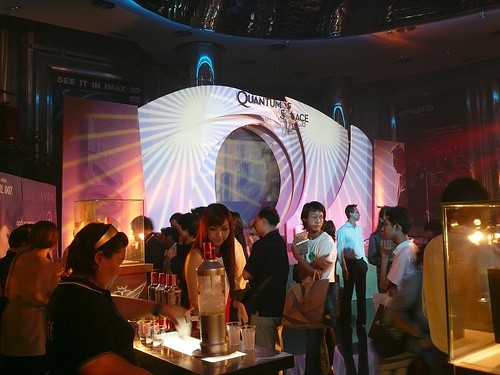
top-left (368, 296), bottom-right (406, 346)
top-left (282, 268), bottom-right (329, 323)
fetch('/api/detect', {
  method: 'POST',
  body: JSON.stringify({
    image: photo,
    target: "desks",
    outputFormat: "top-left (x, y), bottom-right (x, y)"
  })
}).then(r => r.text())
top-left (132, 339), bottom-right (294, 375)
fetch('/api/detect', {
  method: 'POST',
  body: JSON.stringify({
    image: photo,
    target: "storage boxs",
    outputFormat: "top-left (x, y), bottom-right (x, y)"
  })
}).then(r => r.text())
top-left (74, 198), bottom-right (145, 265)
top-left (439, 201), bottom-right (500, 375)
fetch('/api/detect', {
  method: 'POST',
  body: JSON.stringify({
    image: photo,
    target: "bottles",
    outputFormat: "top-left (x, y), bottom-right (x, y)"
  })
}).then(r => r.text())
top-left (162, 274), bottom-right (173, 305)
top-left (147, 272), bottom-right (158, 302)
top-left (167, 274), bottom-right (182, 305)
top-left (196, 242), bottom-right (229, 355)
top-left (155, 273), bottom-right (165, 304)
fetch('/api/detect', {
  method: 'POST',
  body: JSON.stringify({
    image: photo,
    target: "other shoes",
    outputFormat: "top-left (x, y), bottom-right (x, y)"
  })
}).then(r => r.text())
top-left (341, 324), bottom-right (352, 333)
top-left (357, 323), bottom-right (364, 329)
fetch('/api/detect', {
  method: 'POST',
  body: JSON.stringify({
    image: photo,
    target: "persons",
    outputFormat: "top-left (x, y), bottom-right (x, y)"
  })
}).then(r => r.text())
top-left (292, 201), bottom-right (338, 375)
top-left (158, 200), bottom-right (252, 341)
top-left (128, 216), bottom-right (165, 285)
top-left (422, 177), bottom-right (500, 375)
top-left (336, 204), bottom-right (368, 334)
top-left (367, 206), bottom-right (442, 298)
top-left (0, 220), bottom-right (70, 375)
top-left (44, 221), bottom-right (152, 375)
top-left (242, 205), bottom-right (290, 352)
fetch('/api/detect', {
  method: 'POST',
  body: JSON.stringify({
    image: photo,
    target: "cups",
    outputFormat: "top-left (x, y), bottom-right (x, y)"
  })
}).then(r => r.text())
top-left (137, 320), bottom-right (167, 349)
top-left (226, 322), bottom-right (241, 346)
top-left (240, 325), bottom-right (256, 350)
